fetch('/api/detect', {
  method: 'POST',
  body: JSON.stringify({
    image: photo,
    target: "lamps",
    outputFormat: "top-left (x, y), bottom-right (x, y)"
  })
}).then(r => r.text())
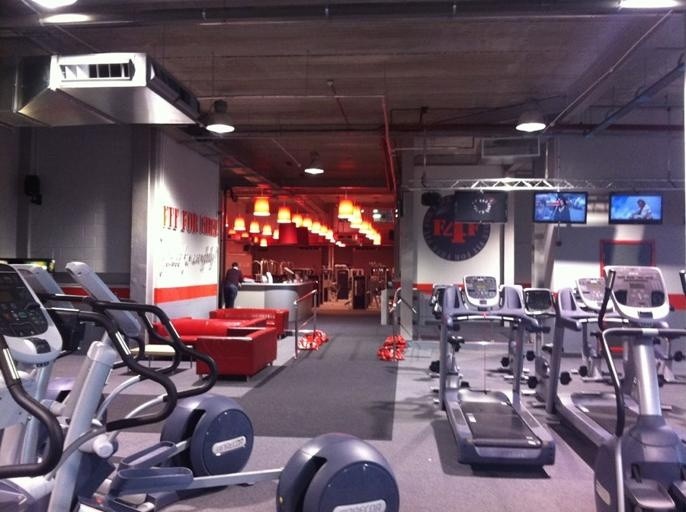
top-left (515, 98), bottom-right (547, 132)
top-left (304, 158), bottom-right (325, 175)
top-left (229, 187), bottom-right (382, 247)
top-left (205, 99), bottom-right (236, 134)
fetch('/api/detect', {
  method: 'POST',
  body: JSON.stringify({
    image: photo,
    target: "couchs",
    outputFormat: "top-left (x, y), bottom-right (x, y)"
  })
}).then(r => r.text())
top-left (196, 326), bottom-right (278, 383)
top-left (209, 308), bottom-right (289, 337)
top-left (150, 316), bottom-right (267, 346)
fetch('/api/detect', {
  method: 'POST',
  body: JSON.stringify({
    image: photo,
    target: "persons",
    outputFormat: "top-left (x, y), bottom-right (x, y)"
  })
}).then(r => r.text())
top-left (223, 262), bottom-right (243, 308)
top-left (629, 199), bottom-right (652, 220)
top-left (551, 197), bottom-right (571, 221)
top-left (535, 199), bottom-right (552, 221)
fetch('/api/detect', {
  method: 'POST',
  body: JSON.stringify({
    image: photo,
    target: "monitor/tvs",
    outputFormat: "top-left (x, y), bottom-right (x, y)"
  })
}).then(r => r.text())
top-left (532, 192), bottom-right (589, 225)
top-left (453, 192), bottom-right (507, 223)
top-left (608, 193), bottom-right (664, 226)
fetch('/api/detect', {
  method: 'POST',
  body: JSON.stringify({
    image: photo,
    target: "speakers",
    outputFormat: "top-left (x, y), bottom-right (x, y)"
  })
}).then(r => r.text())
top-left (480, 137), bottom-right (544, 160)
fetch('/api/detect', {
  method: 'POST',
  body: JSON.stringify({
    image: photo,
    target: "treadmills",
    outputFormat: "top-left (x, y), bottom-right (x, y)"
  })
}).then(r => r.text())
top-left (429, 275), bottom-right (556, 469)
top-left (544, 278), bottom-right (670, 447)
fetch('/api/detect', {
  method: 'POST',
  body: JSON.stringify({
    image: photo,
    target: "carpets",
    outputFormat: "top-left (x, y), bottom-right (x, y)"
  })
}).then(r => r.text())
top-left (55, 336), bottom-right (399, 442)
